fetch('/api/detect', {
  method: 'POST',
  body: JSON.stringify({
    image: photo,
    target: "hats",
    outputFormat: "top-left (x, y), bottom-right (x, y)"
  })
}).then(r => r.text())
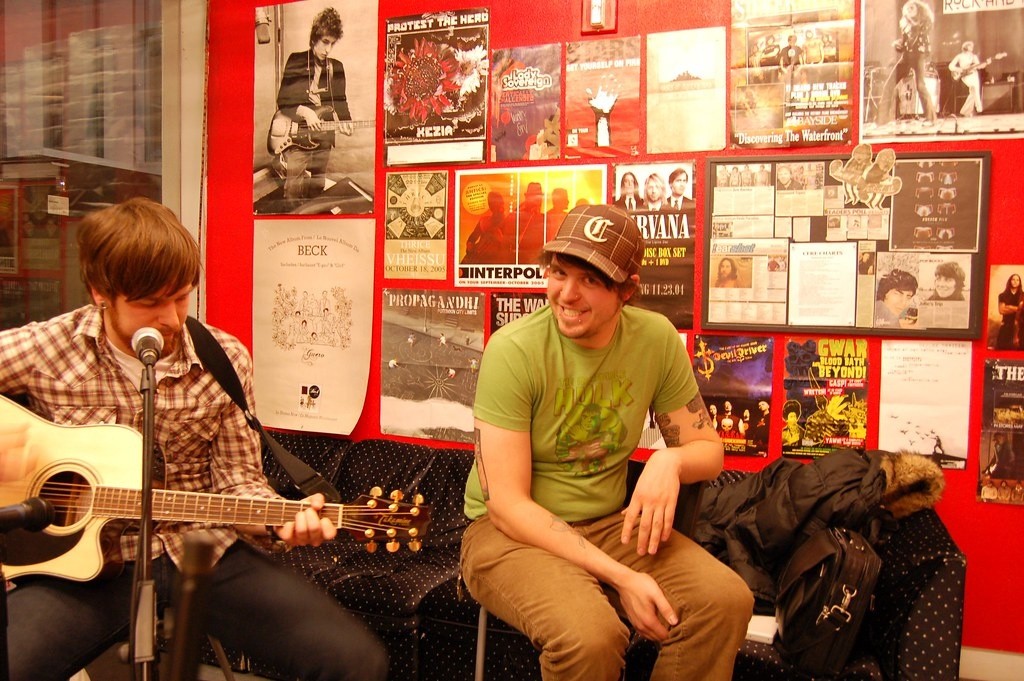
top-left (542, 204), bottom-right (646, 284)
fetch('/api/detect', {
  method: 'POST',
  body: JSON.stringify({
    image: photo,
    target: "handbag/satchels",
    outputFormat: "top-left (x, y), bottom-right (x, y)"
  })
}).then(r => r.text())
top-left (773, 526), bottom-right (883, 675)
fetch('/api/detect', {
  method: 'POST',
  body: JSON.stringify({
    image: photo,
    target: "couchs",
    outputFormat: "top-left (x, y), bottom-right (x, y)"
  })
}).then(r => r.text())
top-left (171, 431), bottom-right (965, 680)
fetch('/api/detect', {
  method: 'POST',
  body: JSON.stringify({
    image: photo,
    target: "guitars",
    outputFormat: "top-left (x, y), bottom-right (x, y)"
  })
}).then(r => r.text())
top-left (950, 51), bottom-right (1007, 83)
top-left (0, 393), bottom-right (434, 585)
top-left (267, 104), bottom-right (376, 156)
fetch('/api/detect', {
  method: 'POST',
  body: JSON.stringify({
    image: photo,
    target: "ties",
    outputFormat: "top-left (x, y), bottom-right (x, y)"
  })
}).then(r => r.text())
top-left (674, 199), bottom-right (679, 211)
top-left (629, 198), bottom-right (633, 210)
top-left (653, 208), bottom-right (658, 210)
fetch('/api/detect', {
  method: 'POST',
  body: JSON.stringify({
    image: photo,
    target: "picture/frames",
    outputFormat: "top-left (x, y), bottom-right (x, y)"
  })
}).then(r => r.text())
top-left (701, 149), bottom-right (990, 341)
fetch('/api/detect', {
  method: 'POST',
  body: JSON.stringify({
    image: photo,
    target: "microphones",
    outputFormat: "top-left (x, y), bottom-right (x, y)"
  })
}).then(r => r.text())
top-left (131, 327), bottom-right (165, 366)
top-left (0, 498), bottom-right (56, 535)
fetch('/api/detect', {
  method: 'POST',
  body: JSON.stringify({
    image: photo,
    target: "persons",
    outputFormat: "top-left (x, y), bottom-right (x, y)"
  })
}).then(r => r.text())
top-left (717, 164), bottom-right (807, 190)
top-left (389, 358), bottom-right (398, 368)
top-left (927, 262), bottom-right (965, 300)
top-left (859, 252), bottom-right (874, 275)
top-left (874, 269), bottom-right (918, 328)
top-left (708, 400), bottom-right (770, 456)
top-left (0, 196), bottom-right (389, 681)
top-left (782, 401), bottom-right (805, 447)
top-left (408, 332), bottom-right (416, 347)
top-left (466, 161), bottom-right (695, 264)
top-left (714, 258), bottom-right (738, 288)
top-left (276, 7), bottom-right (355, 213)
top-left (446, 367), bottom-right (455, 379)
top-left (981, 432), bottom-right (1024, 501)
top-left (461, 204), bottom-right (755, 681)
top-left (760, 1), bottom-right (992, 134)
top-left (437, 333), bottom-right (448, 349)
top-left (996, 274), bottom-right (1024, 349)
top-left (767, 256), bottom-right (787, 272)
top-left (467, 357), bottom-right (479, 373)
